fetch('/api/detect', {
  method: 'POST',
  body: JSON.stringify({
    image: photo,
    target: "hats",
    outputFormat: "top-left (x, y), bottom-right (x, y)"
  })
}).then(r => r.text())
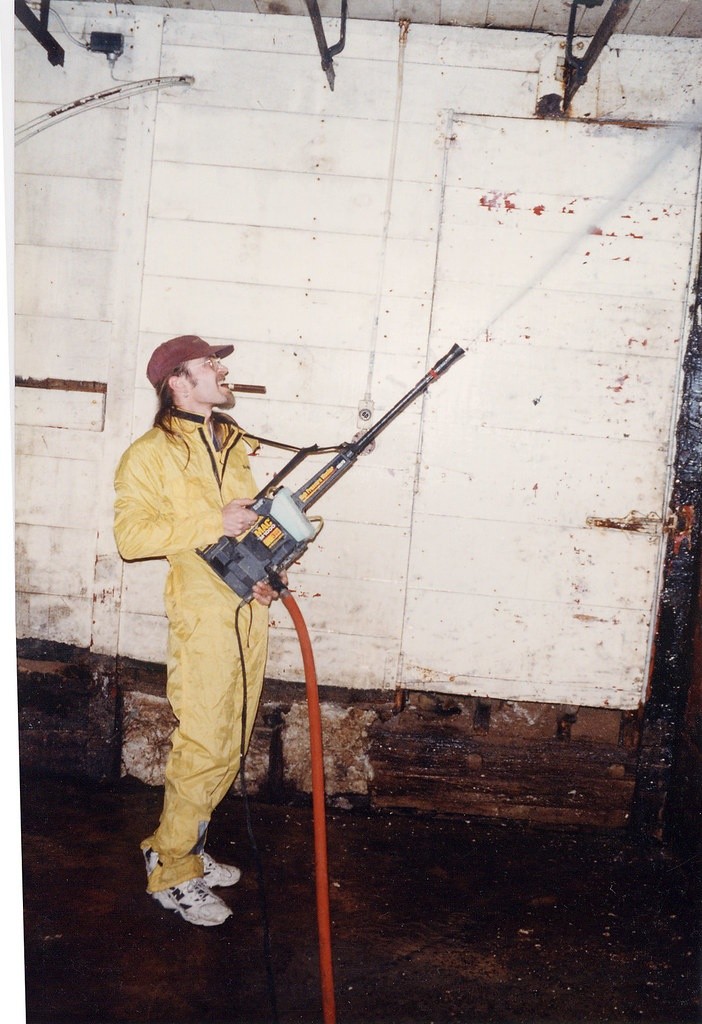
top-left (146, 335), bottom-right (235, 388)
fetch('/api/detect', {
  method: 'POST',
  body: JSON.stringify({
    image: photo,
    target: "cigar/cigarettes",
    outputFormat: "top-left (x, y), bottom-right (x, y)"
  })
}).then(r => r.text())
top-left (221, 383), bottom-right (266, 393)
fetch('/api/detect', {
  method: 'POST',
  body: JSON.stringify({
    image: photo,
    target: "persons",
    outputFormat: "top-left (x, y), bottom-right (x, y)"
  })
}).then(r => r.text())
top-left (112, 334), bottom-right (288, 928)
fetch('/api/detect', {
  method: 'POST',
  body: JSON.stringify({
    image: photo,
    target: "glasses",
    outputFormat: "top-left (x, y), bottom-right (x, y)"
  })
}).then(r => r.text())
top-left (193, 357), bottom-right (221, 367)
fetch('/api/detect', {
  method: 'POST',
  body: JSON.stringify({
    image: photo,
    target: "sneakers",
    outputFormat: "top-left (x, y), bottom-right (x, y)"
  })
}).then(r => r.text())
top-left (146, 878), bottom-right (236, 930)
top-left (143, 848), bottom-right (241, 889)
